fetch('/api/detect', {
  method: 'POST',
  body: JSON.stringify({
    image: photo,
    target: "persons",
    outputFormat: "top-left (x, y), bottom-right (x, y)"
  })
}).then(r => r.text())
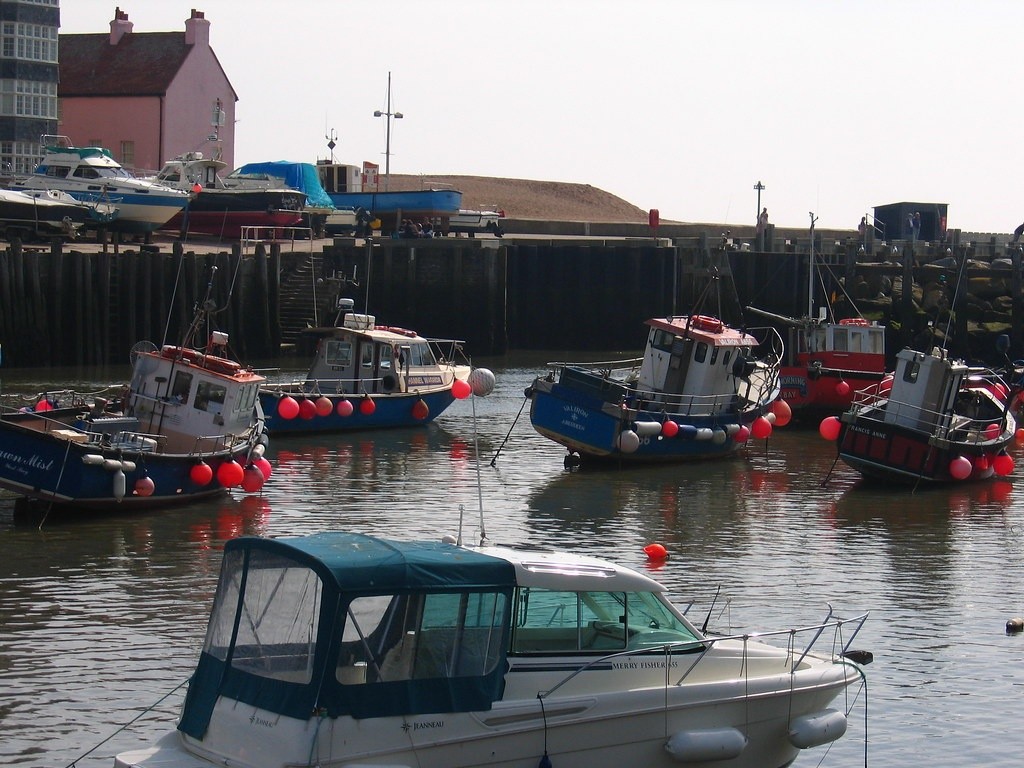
top-left (905, 211), bottom-right (921, 240)
top-left (858, 217), bottom-right (868, 241)
top-left (399, 216), bottom-right (433, 238)
top-left (1013, 224), bottom-right (1024, 242)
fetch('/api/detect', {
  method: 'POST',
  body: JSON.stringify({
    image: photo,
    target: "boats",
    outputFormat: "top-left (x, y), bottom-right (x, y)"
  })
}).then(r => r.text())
top-left (523, 311), bottom-right (785, 461)
top-left (0, 169), bottom-right (123, 238)
top-left (431, 208), bottom-right (502, 231)
top-left (6, 134), bottom-right (192, 233)
top-left (204, 299), bottom-right (472, 437)
top-left (112, 530), bottom-right (874, 767)
top-left (127, 153), bottom-right (309, 236)
top-left (753, 216), bottom-right (898, 416)
top-left (220, 160), bottom-right (370, 234)
top-left (838, 345), bottom-right (1019, 492)
top-left (1, 332), bottom-right (268, 512)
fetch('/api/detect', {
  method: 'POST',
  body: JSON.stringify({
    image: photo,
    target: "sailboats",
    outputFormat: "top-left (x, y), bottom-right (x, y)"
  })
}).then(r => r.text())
top-left (318, 72), bottom-right (465, 233)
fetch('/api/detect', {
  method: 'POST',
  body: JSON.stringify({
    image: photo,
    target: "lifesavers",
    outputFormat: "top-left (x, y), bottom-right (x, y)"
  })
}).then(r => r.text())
top-left (693, 320), bottom-right (723, 332)
top-left (198, 354), bottom-right (241, 376)
top-left (692, 315), bottom-right (724, 326)
top-left (389, 326), bottom-right (417, 338)
top-left (839, 318), bottom-right (868, 323)
top-left (374, 326), bottom-right (388, 330)
top-left (161, 344), bottom-right (202, 363)
top-left (840, 323), bottom-right (869, 326)
